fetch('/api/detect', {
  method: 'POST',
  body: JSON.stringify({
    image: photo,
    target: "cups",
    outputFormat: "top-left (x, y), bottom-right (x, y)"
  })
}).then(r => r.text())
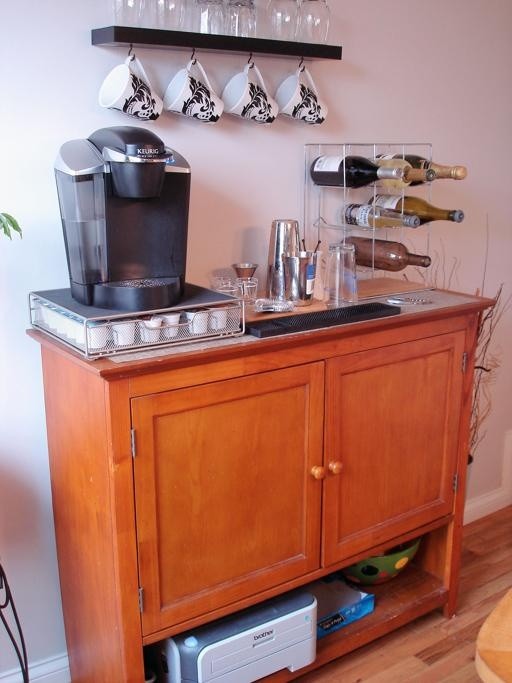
top-left (218, 60), bottom-right (277, 125)
top-left (207, 263), bottom-right (259, 313)
top-left (113, 0), bottom-right (333, 43)
top-left (275, 67), bottom-right (331, 128)
top-left (267, 219), bottom-right (300, 300)
top-left (166, 59), bottom-right (223, 125)
top-left (100, 53), bottom-right (164, 122)
top-left (33, 305), bottom-right (226, 347)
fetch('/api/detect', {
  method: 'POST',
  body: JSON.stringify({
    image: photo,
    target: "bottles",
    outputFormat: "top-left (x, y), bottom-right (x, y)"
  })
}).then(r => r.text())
top-left (342, 202), bottom-right (420, 231)
top-left (283, 243), bottom-right (359, 309)
top-left (312, 154), bottom-right (403, 188)
top-left (369, 193), bottom-right (464, 227)
top-left (376, 152), bottom-right (470, 183)
top-left (373, 155), bottom-right (436, 189)
top-left (340, 236), bottom-right (431, 274)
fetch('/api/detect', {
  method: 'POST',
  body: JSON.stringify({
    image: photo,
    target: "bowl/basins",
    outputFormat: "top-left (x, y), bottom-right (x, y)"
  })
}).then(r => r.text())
top-left (344, 534), bottom-right (422, 587)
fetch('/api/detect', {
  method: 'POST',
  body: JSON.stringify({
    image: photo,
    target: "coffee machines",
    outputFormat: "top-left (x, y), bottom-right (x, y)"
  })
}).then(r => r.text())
top-left (52, 125), bottom-right (191, 311)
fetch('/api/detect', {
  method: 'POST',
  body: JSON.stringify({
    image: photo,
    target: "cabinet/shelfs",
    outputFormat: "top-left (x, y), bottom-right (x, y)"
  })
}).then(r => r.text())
top-left (26, 263), bottom-right (498, 683)
